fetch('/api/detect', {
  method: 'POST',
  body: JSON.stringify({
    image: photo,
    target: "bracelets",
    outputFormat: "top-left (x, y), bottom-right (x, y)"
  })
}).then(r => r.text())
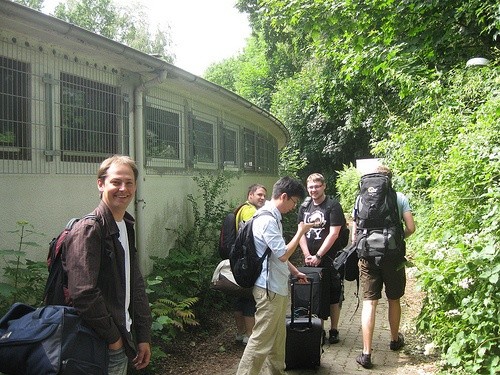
top-left (316, 253), bottom-right (323, 259)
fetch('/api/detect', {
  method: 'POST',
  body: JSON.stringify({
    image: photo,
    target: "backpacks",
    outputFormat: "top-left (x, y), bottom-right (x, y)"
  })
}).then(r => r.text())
top-left (41, 215), bottom-right (106, 307)
top-left (227, 212), bottom-right (279, 299)
top-left (218, 203), bottom-right (250, 260)
top-left (0, 302), bottom-right (109, 375)
top-left (355, 172), bottom-right (406, 272)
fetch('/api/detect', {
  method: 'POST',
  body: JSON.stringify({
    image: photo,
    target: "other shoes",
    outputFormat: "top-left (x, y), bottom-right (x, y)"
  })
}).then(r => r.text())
top-left (242, 337), bottom-right (249, 345)
top-left (356, 351), bottom-right (371, 368)
top-left (389, 331), bottom-right (404, 351)
top-left (328, 329), bottom-right (340, 344)
top-left (321, 329), bottom-right (327, 346)
top-left (236, 333), bottom-right (246, 341)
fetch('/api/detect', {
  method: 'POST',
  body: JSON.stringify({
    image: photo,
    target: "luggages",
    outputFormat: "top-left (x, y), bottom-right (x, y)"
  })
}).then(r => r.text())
top-left (290, 263), bottom-right (332, 320)
top-left (285, 276), bottom-right (323, 371)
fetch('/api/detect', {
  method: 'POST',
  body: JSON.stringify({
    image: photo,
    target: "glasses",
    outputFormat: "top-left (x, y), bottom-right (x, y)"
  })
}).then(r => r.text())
top-left (307, 183), bottom-right (324, 189)
top-left (288, 195), bottom-right (298, 207)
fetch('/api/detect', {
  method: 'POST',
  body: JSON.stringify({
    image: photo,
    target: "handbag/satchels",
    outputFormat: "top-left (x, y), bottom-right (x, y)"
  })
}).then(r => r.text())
top-left (346, 248), bottom-right (359, 295)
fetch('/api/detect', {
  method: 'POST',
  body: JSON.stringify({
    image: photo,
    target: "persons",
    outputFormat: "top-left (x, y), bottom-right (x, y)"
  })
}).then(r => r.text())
top-left (352, 168), bottom-right (415, 369)
top-left (296, 174), bottom-right (345, 344)
top-left (62, 154), bottom-right (151, 375)
top-left (235, 177), bottom-right (314, 375)
top-left (233, 184), bottom-right (267, 345)
